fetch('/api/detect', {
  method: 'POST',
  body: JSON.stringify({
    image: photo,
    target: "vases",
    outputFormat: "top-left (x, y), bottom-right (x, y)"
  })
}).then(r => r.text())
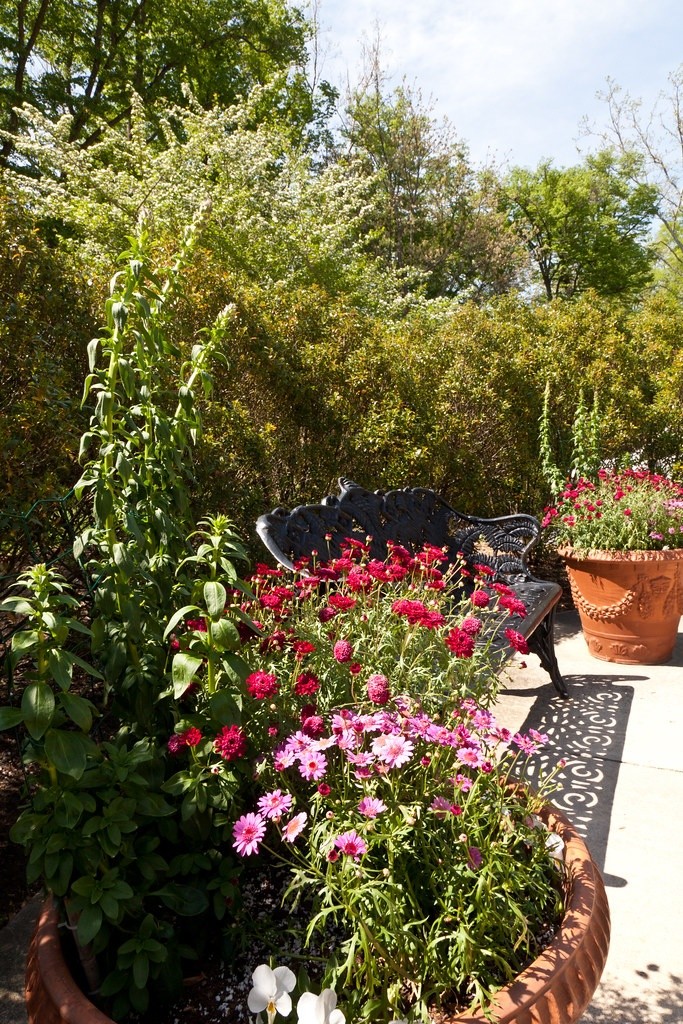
top-left (25, 773), bottom-right (611, 1024)
top-left (558, 547), bottom-right (683, 663)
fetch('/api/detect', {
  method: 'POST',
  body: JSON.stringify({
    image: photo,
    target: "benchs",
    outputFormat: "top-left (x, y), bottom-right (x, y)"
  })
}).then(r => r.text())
top-left (256, 476), bottom-right (572, 697)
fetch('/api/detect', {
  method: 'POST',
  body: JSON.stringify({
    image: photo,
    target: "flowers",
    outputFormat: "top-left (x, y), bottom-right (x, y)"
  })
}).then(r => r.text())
top-left (541, 467), bottom-right (683, 549)
top-left (170, 532), bottom-right (567, 1024)
